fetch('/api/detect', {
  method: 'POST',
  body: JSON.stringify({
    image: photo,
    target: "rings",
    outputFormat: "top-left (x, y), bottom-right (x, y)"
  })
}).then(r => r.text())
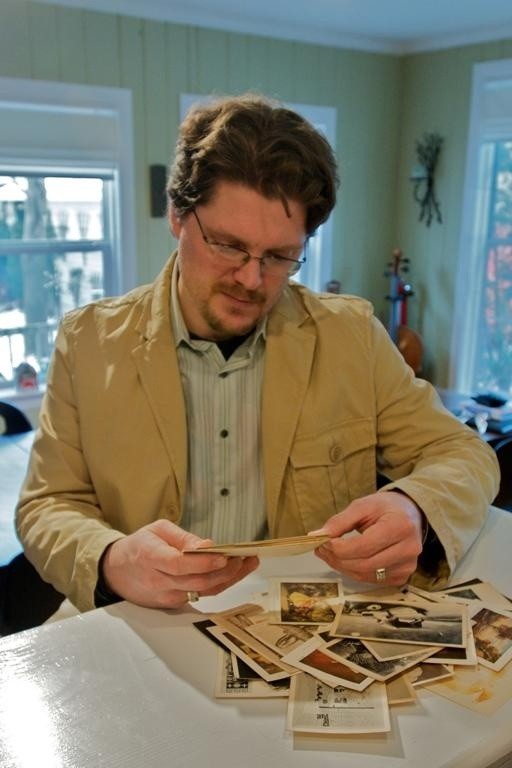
top-left (376, 568), bottom-right (386, 587)
top-left (186, 591), bottom-right (199, 603)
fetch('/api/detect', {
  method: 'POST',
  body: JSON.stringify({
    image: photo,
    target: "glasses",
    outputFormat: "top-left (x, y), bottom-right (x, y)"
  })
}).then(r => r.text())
top-left (192, 203), bottom-right (306, 278)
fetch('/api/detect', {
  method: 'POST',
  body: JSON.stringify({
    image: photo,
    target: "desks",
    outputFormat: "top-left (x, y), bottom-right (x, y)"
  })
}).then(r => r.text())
top-left (0, 504), bottom-right (512, 766)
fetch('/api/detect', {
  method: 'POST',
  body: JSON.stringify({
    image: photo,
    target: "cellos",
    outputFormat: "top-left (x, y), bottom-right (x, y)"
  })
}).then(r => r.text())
top-left (383, 248), bottom-right (423, 377)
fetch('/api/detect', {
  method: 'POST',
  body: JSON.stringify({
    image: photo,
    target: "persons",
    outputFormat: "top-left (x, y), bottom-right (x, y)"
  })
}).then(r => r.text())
top-left (14, 95), bottom-right (501, 617)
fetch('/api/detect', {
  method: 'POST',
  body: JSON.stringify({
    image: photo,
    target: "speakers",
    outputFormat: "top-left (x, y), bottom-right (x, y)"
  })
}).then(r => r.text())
top-left (151, 165), bottom-right (167, 217)
